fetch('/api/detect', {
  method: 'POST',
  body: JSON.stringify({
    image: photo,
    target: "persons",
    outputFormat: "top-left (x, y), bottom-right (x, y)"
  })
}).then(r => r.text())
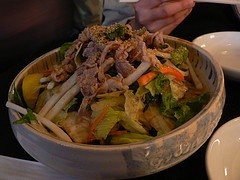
top-left (73, 1), bottom-right (197, 41)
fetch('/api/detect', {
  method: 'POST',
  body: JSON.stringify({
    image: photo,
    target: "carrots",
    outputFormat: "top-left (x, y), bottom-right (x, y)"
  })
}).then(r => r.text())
top-left (138, 71), bottom-right (157, 85)
top-left (160, 64), bottom-right (184, 81)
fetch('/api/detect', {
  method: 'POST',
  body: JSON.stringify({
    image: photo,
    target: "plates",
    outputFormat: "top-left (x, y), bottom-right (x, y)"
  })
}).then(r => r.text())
top-left (193, 32), bottom-right (240, 78)
top-left (206, 116), bottom-right (240, 180)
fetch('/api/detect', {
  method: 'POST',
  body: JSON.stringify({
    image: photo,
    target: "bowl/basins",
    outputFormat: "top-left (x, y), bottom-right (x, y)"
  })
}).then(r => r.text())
top-left (9, 34), bottom-right (226, 180)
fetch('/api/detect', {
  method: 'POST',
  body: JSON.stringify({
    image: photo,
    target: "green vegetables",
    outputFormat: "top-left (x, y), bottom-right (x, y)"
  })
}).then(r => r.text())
top-left (10, 23), bottom-right (212, 145)
